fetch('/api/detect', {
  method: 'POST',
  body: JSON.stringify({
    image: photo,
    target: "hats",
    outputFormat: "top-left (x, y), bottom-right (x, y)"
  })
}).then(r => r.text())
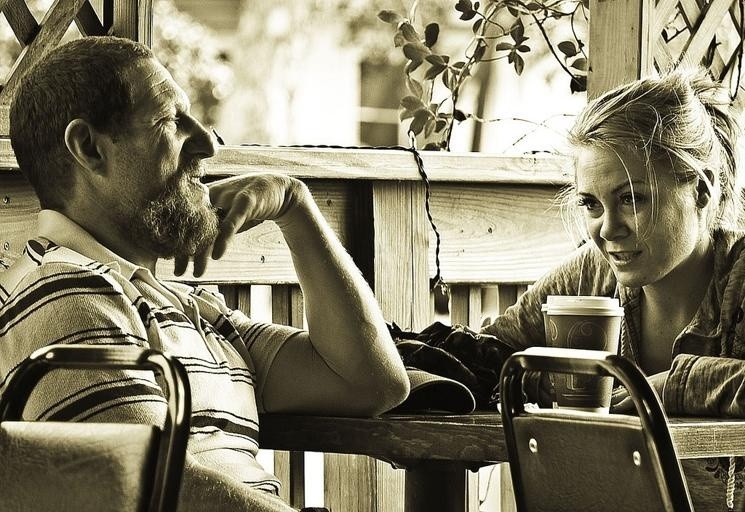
top-left (404, 366), bottom-right (476, 415)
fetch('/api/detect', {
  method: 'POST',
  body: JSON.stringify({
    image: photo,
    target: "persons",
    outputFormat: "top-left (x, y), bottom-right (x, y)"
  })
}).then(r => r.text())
top-left (0, 32), bottom-right (412, 511)
top-left (458, 66), bottom-right (744, 439)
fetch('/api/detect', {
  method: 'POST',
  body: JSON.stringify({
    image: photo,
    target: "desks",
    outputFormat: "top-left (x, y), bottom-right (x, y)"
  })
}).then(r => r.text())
top-left (257, 409), bottom-right (745, 512)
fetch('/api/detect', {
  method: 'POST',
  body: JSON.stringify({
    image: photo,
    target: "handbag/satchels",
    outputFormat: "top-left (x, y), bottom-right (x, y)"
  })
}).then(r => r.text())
top-left (387, 322), bottom-right (515, 409)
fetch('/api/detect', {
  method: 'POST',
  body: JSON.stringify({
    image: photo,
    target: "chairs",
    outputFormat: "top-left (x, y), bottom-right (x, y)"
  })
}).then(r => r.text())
top-left (0, 342), bottom-right (194, 512)
top-left (498, 345), bottom-right (696, 512)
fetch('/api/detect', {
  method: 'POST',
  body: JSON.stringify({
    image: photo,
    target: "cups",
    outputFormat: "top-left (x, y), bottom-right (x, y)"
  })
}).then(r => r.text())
top-left (541, 294), bottom-right (625, 416)
